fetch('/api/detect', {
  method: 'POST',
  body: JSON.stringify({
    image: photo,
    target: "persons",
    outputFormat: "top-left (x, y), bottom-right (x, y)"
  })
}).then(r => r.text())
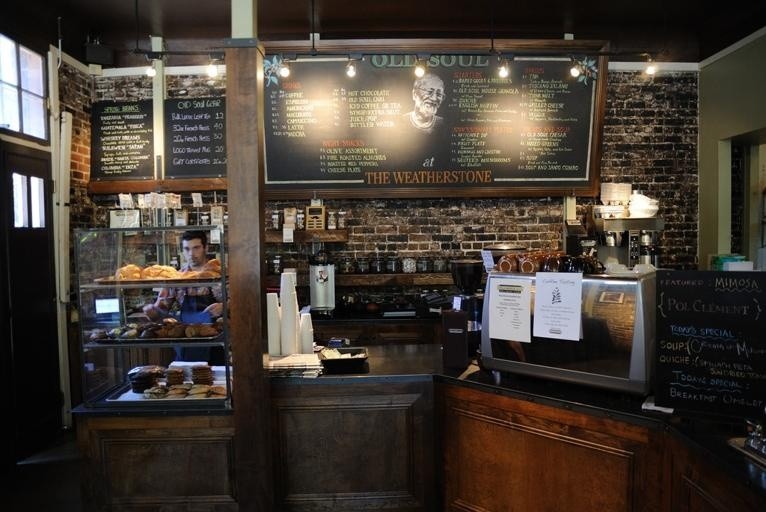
top-left (401, 72), bottom-right (448, 145)
top-left (144, 230), bottom-right (230, 364)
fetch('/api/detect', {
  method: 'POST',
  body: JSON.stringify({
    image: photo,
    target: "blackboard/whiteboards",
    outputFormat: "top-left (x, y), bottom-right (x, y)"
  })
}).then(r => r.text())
top-left (655, 269), bottom-right (766, 420)
top-left (91, 95), bottom-right (227, 179)
top-left (257, 39), bottom-right (609, 200)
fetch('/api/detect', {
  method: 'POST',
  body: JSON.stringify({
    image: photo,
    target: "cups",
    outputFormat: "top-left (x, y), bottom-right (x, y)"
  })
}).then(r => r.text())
top-left (266, 273), bottom-right (314, 356)
top-left (497, 254), bottom-right (606, 276)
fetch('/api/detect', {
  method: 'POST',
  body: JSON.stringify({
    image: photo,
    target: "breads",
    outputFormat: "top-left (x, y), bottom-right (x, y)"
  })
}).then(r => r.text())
top-left (97, 318), bottom-right (225, 338)
top-left (127, 366), bottom-right (226, 399)
top-left (110, 258), bottom-right (222, 280)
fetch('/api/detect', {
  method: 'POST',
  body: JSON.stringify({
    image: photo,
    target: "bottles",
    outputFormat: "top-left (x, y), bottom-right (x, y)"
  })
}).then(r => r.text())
top-left (273, 254), bottom-right (282, 274)
top-left (271, 209), bottom-right (347, 230)
top-left (744, 425), bottom-right (766, 492)
top-left (339, 256), bottom-right (458, 274)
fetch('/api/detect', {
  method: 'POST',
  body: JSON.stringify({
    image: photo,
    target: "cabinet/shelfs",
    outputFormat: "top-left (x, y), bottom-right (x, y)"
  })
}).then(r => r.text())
top-left (72, 226), bottom-right (231, 410)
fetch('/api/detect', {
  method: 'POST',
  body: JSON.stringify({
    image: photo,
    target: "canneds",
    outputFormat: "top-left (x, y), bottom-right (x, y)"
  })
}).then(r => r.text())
top-left (433, 258), bottom-right (448, 273)
top-left (339, 258), bottom-right (357, 274)
top-left (447, 258), bottom-right (458, 272)
top-left (385, 256), bottom-right (401, 273)
top-left (356, 257), bottom-right (370, 274)
top-left (416, 257), bottom-right (434, 273)
top-left (370, 259), bottom-right (385, 273)
top-left (401, 257), bottom-right (417, 274)
